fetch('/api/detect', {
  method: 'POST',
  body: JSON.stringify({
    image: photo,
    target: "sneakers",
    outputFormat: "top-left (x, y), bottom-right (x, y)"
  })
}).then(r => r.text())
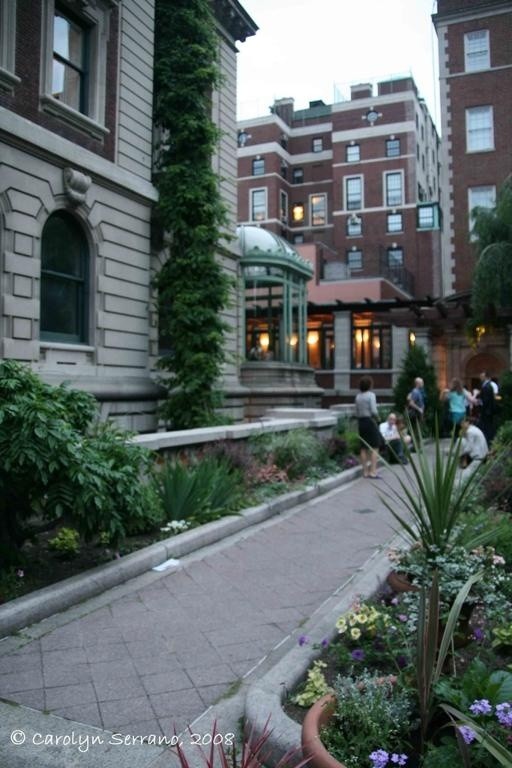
top-left (362, 472), bottom-right (382, 480)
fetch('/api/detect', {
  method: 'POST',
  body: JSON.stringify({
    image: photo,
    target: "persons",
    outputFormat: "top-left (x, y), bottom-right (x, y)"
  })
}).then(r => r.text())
top-left (248, 340), bottom-right (262, 360)
top-left (355, 375), bottom-right (381, 478)
top-left (438, 369), bottom-right (503, 471)
top-left (407, 376), bottom-right (425, 453)
top-left (378, 412), bottom-right (407, 464)
top-left (395, 415), bottom-right (412, 452)
top-left (262, 346), bottom-right (275, 360)
